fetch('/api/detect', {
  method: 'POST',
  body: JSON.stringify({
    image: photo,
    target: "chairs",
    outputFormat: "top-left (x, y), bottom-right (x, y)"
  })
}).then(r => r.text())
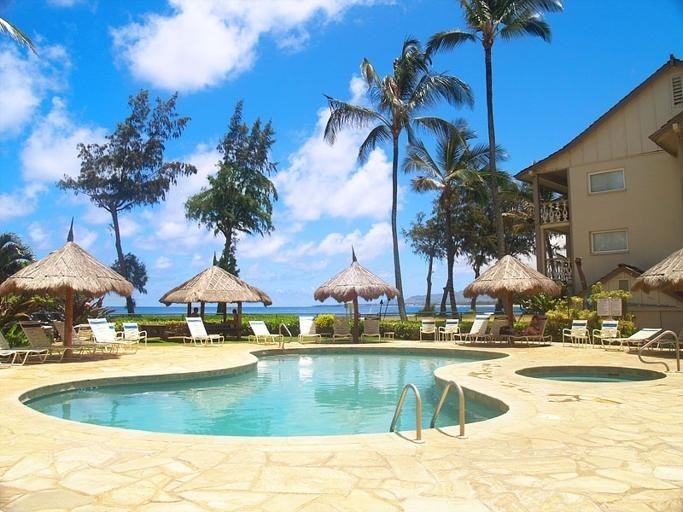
top-left (0, 318), bottom-right (147, 366)
top-left (298, 316), bottom-right (321, 344)
top-left (420, 314), bottom-right (551, 347)
top-left (333, 316), bottom-right (353, 343)
top-left (248, 320), bottom-right (283, 344)
top-left (185, 317), bottom-right (224, 347)
top-left (563, 320), bottom-right (662, 353)
top-left (361, 320), bottom-right (381, 343)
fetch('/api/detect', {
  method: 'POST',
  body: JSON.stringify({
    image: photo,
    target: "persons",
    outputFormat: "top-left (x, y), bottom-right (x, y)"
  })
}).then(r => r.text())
top-left (223, 308), bottom-right (237, 328)
top-left (518, 316), bottom-right (541, 337)
top-left (190, 307), bottom-right (198, 317)
